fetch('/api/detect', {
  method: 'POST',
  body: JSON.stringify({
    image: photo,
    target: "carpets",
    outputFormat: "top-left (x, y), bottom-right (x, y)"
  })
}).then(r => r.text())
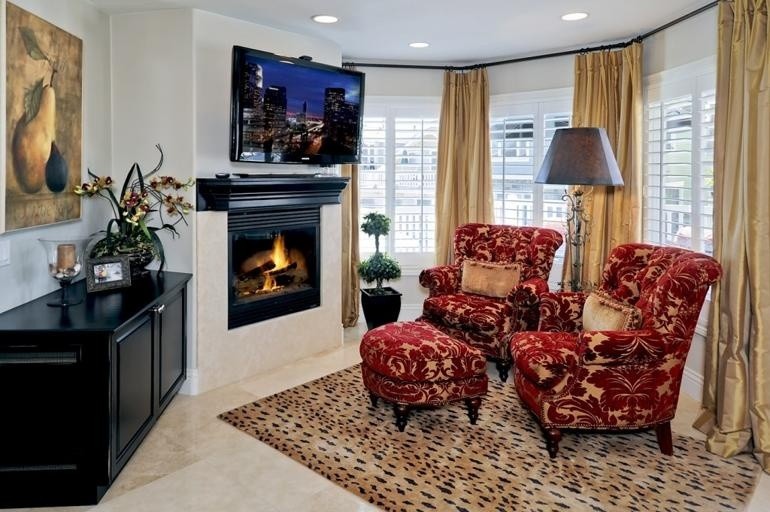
top-left (215, 360), bottom-right (761, 512)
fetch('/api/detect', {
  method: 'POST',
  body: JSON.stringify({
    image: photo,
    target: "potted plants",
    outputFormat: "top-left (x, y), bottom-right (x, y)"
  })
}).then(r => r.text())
top-left (357, 213), bottom-right (403, 331)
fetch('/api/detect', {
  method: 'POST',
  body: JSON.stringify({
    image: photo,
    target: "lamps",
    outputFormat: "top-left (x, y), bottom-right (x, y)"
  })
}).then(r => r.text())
top-left (536, 128), bottom-right (627, 291)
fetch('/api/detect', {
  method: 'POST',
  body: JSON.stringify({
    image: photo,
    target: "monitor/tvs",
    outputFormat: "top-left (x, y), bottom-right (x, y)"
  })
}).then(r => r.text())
top-left (229, 45), bottom-right (366, 165)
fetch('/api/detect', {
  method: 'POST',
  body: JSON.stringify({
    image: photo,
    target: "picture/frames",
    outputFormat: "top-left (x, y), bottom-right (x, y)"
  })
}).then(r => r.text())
top-left (86, 256), bottom-right (135, 294)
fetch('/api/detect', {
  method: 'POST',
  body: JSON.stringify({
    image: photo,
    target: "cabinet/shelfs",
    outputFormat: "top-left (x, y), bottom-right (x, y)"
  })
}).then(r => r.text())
top-left (1, 269), bottom-right (193, 506)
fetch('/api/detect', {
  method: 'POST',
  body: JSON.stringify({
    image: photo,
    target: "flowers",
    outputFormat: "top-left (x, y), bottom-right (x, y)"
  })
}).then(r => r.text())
top-left (74, 144), bottom-right (194, 278)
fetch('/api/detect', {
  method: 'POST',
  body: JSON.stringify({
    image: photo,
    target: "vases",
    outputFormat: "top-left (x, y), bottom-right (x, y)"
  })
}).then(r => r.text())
top-left (90, 232), bottom-right (158, 274)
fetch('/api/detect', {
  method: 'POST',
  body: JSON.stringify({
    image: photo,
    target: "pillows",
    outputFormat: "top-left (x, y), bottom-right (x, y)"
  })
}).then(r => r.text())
top-left (461, 260), bottom-right (523, 298)
top-left (581, 287), bottom-right (645, 331)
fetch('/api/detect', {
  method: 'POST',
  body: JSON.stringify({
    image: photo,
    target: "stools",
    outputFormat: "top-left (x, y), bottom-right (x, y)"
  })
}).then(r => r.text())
top-left (362, 320), bottom-right (489, 431)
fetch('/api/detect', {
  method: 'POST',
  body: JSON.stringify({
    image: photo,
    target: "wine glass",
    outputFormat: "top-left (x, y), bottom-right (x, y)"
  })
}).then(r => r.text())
top-left (38, 235), bottom-right (93, 308)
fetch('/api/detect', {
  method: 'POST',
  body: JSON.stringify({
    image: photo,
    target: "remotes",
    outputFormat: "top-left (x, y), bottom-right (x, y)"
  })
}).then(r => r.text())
top-left (216, 173), bottom-right (230, 179)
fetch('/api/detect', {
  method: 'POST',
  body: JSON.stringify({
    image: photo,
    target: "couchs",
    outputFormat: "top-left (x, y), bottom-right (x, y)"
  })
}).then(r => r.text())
top-left (417, 223), bottom-right (562, 382)
top-left (513, 243), bottom-right (723, 458)
